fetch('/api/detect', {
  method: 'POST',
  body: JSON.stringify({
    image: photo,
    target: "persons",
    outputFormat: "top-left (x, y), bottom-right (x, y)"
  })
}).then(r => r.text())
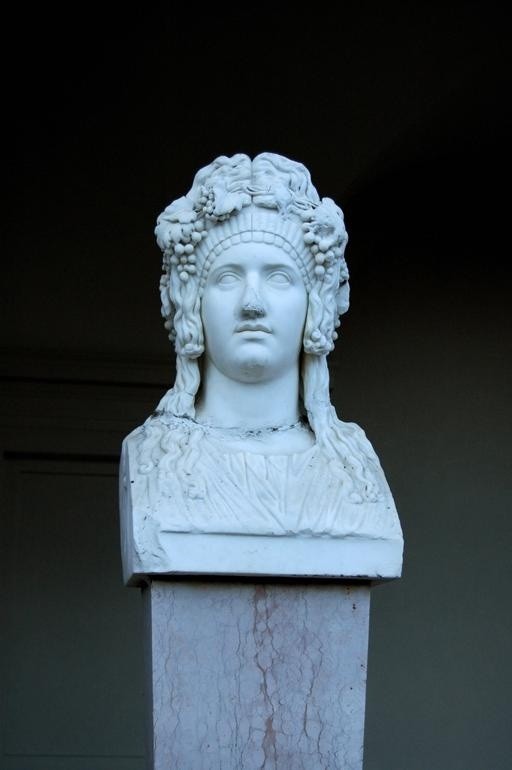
top-left (118, 152), bottom-right (407, 584)
